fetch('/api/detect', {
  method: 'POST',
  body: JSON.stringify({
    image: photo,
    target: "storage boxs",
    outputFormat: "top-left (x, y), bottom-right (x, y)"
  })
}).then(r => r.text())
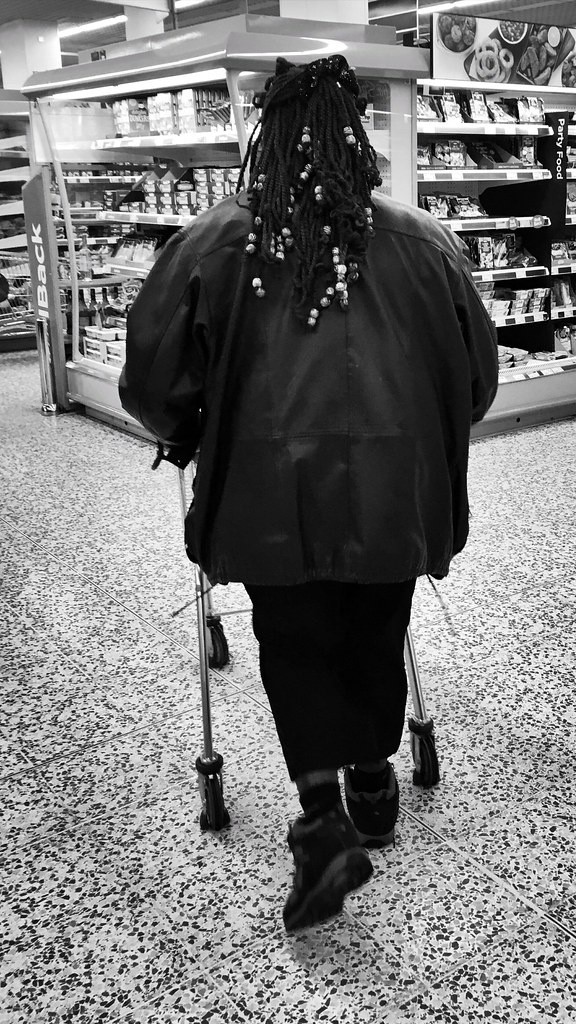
top-left (463, 236), bottom-right (550, 317)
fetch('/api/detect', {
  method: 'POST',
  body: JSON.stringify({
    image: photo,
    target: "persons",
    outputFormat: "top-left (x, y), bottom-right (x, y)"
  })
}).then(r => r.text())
top-left (120, 54), bottom-right (499, 931)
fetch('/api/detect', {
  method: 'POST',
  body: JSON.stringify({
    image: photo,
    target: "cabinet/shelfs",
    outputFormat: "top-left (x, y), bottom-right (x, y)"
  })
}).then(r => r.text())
top-left (0, 75), bottom-right (390, 370)
top-left (414, 77), bottom-right (576, 442)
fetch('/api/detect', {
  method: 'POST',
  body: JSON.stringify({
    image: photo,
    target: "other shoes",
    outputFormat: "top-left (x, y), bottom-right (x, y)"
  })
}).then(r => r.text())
top-left (343, 760), bottom-right (400, 847)
top-left (283, 805), bottom-right (373, 933)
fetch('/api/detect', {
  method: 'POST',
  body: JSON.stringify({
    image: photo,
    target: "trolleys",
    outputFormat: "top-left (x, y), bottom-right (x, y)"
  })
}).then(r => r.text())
top-left (176, 450), bottom-right (441, 832)
